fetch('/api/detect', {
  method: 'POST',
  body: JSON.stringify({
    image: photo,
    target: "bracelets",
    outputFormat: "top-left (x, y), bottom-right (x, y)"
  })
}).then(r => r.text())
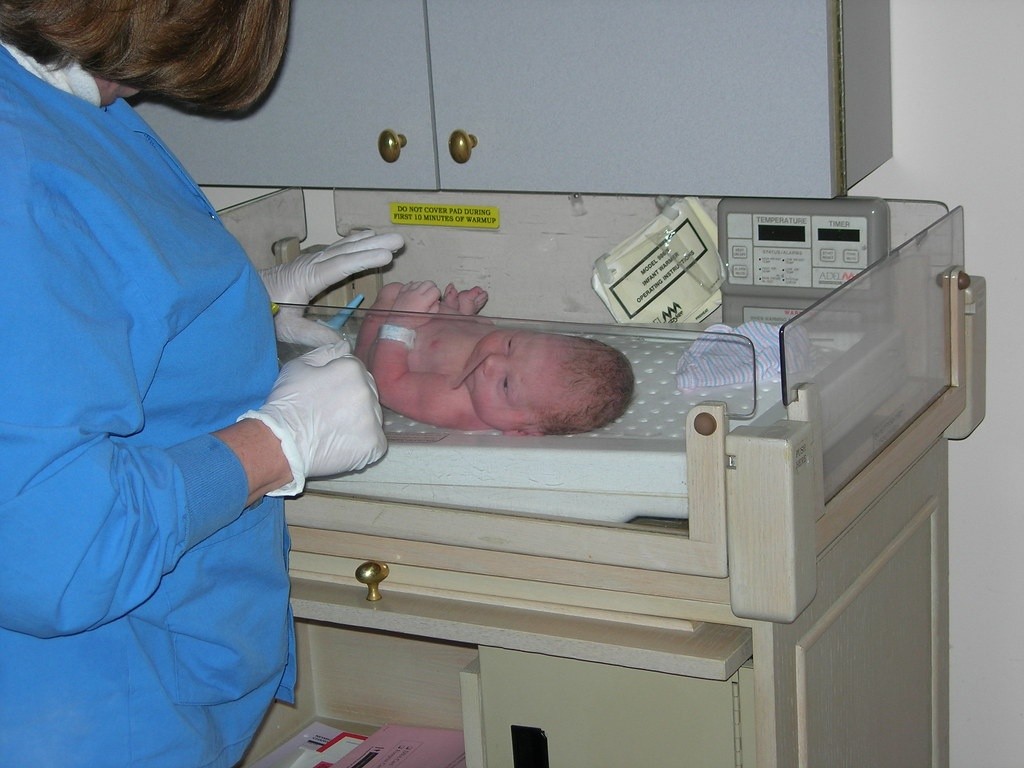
top-left (379, 324), bottom-right (415, 349)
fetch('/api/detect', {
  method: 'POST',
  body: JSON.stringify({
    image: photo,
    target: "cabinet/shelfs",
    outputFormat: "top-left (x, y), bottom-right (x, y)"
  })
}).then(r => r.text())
top-left (128, 0), bottom-right (893, 200)
top-left (475, 645), bottom-right (741, 768)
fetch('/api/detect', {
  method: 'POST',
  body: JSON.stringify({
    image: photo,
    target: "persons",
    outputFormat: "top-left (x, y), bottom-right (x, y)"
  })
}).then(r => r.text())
top-left (0, 0), bottom-right (404, 768)
top-left (352, 280), bottom-right (634, 437)
